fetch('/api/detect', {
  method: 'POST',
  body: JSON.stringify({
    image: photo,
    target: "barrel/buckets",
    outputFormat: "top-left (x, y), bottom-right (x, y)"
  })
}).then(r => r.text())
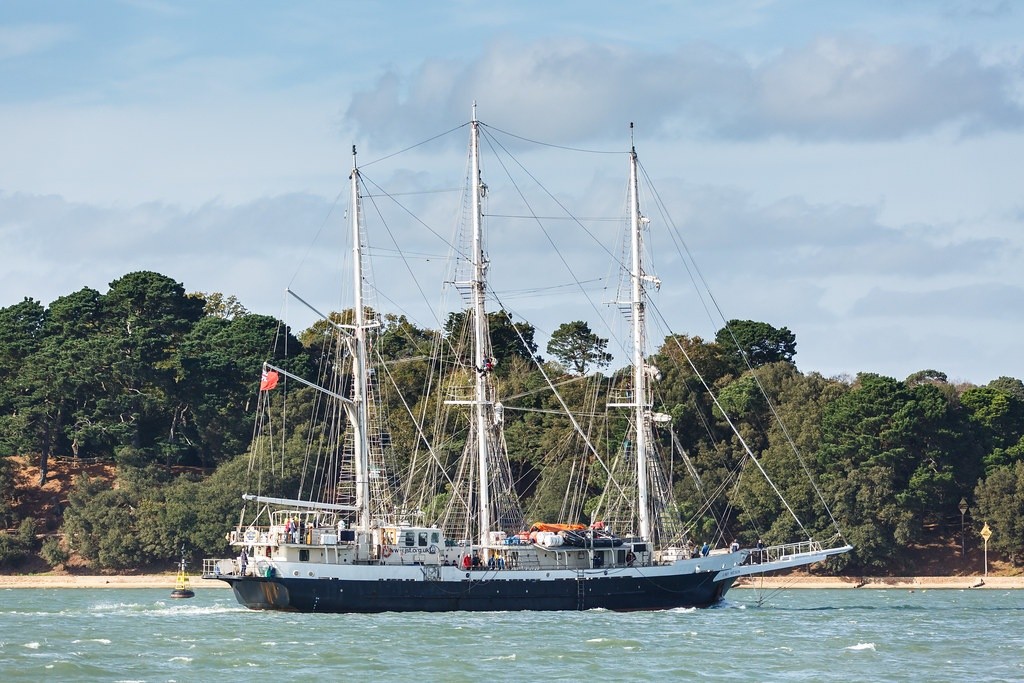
top-left (536, 532), bottom-right (563, 547)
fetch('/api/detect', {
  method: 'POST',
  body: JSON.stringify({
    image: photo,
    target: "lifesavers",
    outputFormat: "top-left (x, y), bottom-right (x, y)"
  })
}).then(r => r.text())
top-left (382, 546), bottom-right (392, 558)
top-left (253, 555), bottom-right (274, 577)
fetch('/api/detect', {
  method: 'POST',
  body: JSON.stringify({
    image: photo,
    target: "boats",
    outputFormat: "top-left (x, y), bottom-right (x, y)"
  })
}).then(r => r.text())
top-left (171, 544), bottom-right (194, 598)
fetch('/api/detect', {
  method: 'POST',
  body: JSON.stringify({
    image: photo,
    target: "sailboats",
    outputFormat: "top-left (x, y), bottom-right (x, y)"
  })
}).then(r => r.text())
top-left (201, 100), bottom-right (853, 614)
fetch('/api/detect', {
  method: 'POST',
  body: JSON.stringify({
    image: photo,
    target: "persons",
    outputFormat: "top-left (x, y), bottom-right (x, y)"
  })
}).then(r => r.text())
top-left (626, 549), bottom-right (636, 567)
top-left (693, 545), bottom-right (699, 557)
top-left (464, 554), bottom-right (505, 570)
top-left (702, 542), bottom-right (709, 557)
top-left (730, 539), bottom-right (739, 553)
top-left (757, 540), bottom-right (763, 549)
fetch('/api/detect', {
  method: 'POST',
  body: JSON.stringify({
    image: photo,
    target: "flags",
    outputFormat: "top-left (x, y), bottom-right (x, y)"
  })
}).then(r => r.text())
top-left (260, 372), bottom-right (279, 390)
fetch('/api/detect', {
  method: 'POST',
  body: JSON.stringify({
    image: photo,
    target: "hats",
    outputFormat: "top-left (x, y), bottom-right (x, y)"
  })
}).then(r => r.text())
top-left (445, 560), bottom-right (449, 562)
top-left (758, 540), bottom-right (761, 543)
top-left (475, 554), bottom-right (478, 555)
top-left (453, 560), bottom-right (456, 562)
top-left (468, 554), bottom-right (470, 557)
